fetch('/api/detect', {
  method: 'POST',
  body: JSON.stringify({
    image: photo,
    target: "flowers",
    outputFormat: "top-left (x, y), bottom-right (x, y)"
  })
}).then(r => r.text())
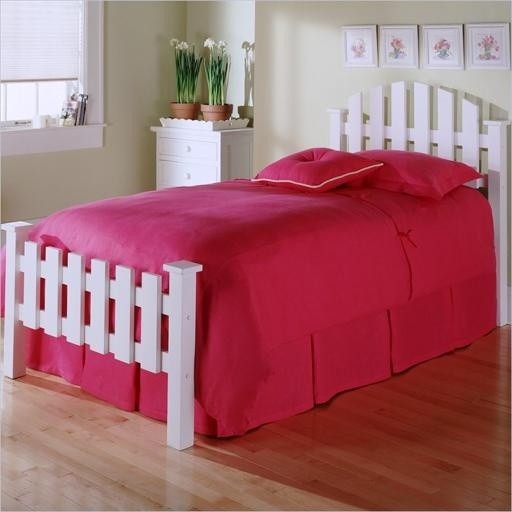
top-left (201, 36), bottom-right (234, 106)
top-left (169, 36), bottom-right (204, 103)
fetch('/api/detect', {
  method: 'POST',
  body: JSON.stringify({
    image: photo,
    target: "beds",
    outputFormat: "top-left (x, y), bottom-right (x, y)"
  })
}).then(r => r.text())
top-left (0, 79), bottom-right (512, 454)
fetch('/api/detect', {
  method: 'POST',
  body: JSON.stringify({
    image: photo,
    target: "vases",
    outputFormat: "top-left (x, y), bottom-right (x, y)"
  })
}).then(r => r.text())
top-left (200, 104), bottom-right (234, 121)
top-left (170, 100), bottom-right (200, 120)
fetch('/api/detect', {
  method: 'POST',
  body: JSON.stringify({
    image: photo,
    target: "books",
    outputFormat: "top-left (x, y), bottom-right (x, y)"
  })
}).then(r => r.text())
top-left (59, 94), bottom-right (88, 126)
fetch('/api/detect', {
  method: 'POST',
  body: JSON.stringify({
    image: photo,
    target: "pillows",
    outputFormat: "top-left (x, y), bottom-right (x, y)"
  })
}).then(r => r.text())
top-left (250, 145), bottom-right (387, 198)
top-left (353, 147), bottom-right (484, 203)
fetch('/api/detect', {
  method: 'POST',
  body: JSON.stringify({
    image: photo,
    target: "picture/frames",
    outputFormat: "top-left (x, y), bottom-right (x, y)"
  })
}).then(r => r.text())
top-left (340, 21), bottom-right (380, 70)
top-left (422, 21), bottom-right (466, 72)
top-left (468, 19), bottom-right (511, 70)
top-left (377, 24), bottom-right (419, 70)
